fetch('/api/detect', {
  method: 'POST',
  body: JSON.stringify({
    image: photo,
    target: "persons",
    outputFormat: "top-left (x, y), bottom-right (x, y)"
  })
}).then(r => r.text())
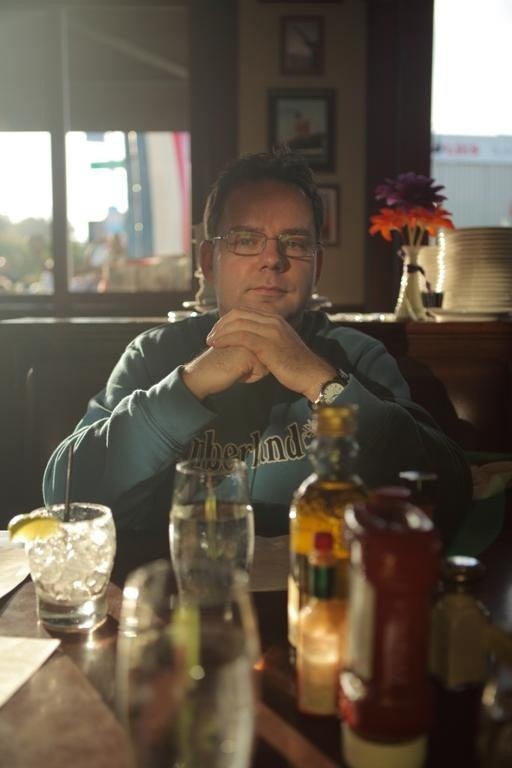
top-left (41, 141), bottom-right (473, 593)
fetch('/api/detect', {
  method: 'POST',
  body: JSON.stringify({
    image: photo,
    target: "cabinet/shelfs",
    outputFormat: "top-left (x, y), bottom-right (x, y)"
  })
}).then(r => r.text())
top-left (2, 314), bottom-right (511, 514)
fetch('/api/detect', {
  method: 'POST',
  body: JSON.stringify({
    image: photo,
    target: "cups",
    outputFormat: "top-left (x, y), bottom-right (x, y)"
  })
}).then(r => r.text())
top-left (117, 560), bottom-right (255, 767)
top-left (27, 506), bottom-right (118, 630)
top-left (169, 459), bottom-right (254, 605)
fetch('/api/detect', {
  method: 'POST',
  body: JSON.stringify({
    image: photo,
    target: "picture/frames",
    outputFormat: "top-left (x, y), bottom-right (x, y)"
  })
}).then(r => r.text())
top-left (313, 184), bottom-right (340, 246)
top-left (279, 13), bottom-right (324, 77)
top-left (267, 87), bottom-right (337, 175)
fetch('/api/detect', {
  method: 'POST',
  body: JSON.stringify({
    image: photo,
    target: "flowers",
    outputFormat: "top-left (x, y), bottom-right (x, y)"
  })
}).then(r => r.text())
top-left (368, 172), bottom-right (454, 246)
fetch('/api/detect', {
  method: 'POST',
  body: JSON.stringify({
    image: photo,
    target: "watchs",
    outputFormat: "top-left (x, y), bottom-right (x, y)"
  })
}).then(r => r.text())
top-left (312, 368), bottom-right (350, 415)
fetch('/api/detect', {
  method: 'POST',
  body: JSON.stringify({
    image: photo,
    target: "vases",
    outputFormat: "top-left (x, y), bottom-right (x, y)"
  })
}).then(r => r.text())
top-left (394, 246), bottom-right (426, 321)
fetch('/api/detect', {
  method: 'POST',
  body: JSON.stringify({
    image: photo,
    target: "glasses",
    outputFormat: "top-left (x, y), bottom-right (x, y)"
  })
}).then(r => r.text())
top-left (210, 228), bottom-right (324, 258)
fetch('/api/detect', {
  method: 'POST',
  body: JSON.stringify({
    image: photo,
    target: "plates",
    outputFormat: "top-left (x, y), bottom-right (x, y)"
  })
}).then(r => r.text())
top-left (439, 316), bottom-right (497, 322)
top-left (415, 243), bottom-right (438, 291)
top-left (439, 228), bottom-right (508, 317)
top-left (430, 307), bottom-right (507, 315)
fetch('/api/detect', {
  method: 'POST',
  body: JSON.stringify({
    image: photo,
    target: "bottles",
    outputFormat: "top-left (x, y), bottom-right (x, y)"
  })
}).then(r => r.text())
top-left (348, 489), bottom-right (431, 765)
top-left (288, 405), bottom-right (365, 713)
top-left (400, 472), bottom-right (442, 526)
top-left (426, 557), bottom-right (493, 729)
